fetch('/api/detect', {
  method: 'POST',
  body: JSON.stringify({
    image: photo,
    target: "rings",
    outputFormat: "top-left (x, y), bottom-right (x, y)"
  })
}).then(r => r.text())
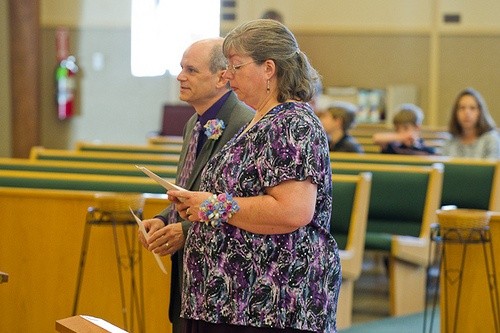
top-left (165, 243), bottom-right (169, 247)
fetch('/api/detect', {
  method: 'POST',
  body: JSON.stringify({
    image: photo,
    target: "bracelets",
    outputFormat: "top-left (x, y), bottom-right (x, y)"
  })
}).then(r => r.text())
top-left (200, 192), bottom-right (240, 227)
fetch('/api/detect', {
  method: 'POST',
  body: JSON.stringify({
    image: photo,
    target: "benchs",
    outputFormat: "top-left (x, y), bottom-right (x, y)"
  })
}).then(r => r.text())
top-left (0, 135), bottom-right (500, 333)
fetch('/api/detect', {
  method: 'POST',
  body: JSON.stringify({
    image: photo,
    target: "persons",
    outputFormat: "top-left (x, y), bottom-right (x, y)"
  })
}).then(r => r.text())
top-left (138, 36), bottom-right (257, 333)
top-left (374, 105), bottom-right (437, 156)
top-left (441, 88), bottom-right (500, 159)
top-left (322, 104), bottom-right (365, 153)
top-left (168, 19), bottom-right (342, 333)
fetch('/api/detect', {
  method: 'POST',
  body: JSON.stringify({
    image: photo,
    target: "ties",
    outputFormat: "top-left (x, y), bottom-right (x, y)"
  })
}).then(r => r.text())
top-left (168, 121), bottom-right (203, 224)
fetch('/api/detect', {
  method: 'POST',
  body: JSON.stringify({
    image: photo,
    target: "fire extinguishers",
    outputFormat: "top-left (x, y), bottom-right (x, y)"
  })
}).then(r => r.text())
top-left (56, 55), bottom-right (78, 120)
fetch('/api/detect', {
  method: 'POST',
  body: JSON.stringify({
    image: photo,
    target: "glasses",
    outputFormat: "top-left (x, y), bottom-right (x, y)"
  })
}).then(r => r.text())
top-left (225, 59), bottom-right (276, 74)
